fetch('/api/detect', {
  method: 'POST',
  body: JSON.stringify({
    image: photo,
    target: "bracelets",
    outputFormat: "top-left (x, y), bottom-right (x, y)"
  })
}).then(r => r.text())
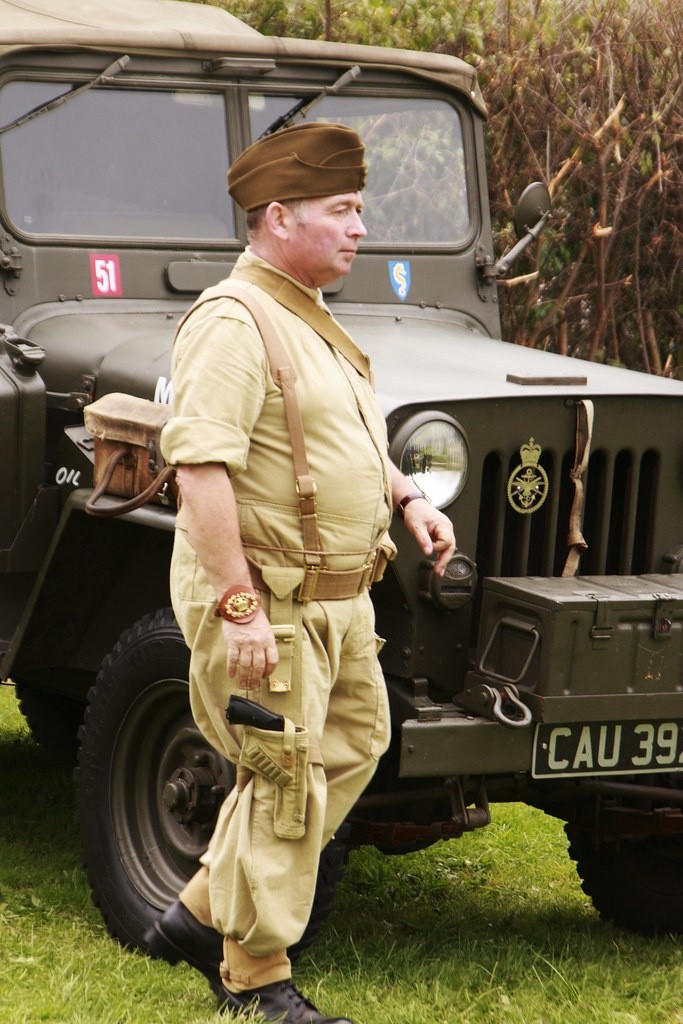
top-left (213, 584), bottom-right (262, 625)
top-left (396, 491), bottom-right (426, 519)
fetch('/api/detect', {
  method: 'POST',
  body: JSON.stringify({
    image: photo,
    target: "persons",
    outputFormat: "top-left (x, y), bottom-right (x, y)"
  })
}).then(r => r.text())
top-left (141, 122), bottom-right (457, 1023)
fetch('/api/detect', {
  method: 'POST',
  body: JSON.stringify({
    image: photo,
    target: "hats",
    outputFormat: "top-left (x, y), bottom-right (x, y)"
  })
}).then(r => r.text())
top-left (227, 122), bottom-right (368, 213)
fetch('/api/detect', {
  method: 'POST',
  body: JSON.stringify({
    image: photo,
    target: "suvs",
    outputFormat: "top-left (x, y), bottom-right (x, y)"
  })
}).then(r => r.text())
top-left (0, 0), bottom-right (682, 965)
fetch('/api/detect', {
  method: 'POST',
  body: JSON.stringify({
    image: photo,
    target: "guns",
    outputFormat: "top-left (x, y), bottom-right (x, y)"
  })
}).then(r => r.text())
top-left (224, 694), bottom-right (285, 732)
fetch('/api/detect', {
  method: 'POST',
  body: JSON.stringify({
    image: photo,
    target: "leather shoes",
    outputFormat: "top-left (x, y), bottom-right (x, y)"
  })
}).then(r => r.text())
top-left (218, 980), bottom-right (357, 1024)
top-left (143, 898), bottom-right (226, 995)
